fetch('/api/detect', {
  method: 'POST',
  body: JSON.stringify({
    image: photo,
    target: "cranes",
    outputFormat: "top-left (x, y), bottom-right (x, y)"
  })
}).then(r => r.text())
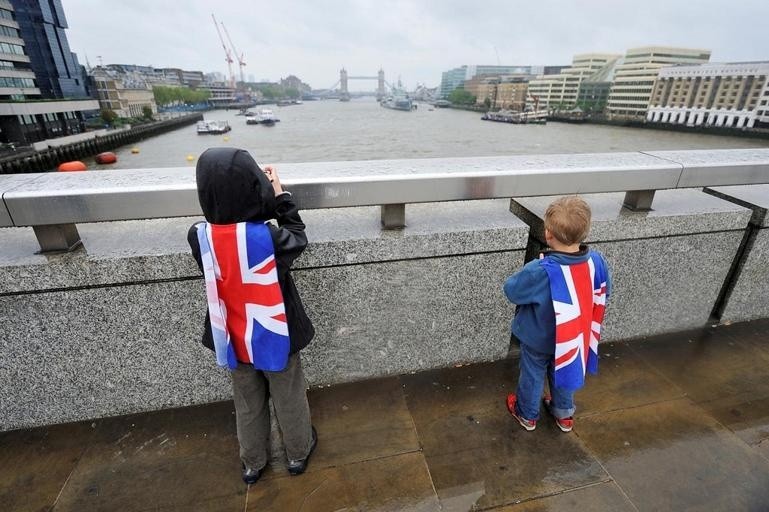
top-left (211, 14), bottom-right (247, 88)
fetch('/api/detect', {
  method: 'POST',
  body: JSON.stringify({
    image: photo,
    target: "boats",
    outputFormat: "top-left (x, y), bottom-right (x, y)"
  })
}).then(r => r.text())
top-left (379, 95), bottom-right (417, 111)
top-left (246, 109), bottom-right (276, 124)
top-left (197, 120), bottom-right (231, 134)
top-left (481, 110), bottom-right (546, 124)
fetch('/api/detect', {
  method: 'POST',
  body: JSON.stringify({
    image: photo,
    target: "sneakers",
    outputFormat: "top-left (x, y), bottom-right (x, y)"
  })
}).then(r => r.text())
top-left (242, 458), bottom-right (262, 485)
top-left (543, 395), bottom-right (574, 434)
top-left (505, 393), bottom-right (537, 433)
top-left (286, 425), bottom-right (318, 476)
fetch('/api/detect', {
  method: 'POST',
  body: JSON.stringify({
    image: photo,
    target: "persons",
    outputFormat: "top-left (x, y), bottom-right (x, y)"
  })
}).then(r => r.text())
top-left (499, 194), bottom-right (614, 433)
top-left (185, 146), bottom-right (320, 487)
